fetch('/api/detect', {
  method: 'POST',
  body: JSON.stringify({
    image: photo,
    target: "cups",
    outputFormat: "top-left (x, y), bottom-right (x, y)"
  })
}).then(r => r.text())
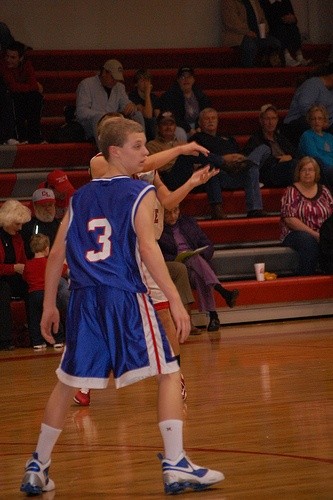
top-left (254, 263), bottom-right (265, 281)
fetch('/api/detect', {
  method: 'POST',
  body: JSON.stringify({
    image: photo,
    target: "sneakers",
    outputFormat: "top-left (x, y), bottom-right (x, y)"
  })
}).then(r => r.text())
top-left (157, 451), bottom-right (225, 495)
top-left (4, 137), bottom-right (47, 145)
top-left (180, 373), bottom-right (188, 400)
top-left (20, 452), bottom-right (52, 493)
top-left (53, 339), bottom-right (64, 348)
top-left (73, 389), bottom-right (90, 406)
top-left (33, 340), bottom-right (47, 348)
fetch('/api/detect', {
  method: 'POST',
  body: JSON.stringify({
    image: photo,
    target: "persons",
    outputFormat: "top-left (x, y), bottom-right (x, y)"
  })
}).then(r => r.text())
top-left (159, 202), bottom-right (240, 335)
top-left (260, 0), bottom-right (312, 67)
top-left (72, 111), bottom-right (220, 404)
top-left (164, 261), bottom-right (202, 335)
top-left (37, 168), bottom-right (78, 219)
top-left (21, 188), bottom-right (74, 302)
top-left (0, 44), bottom-right (45, 146)
top-left (20, 117), bottom-right (228, 493)
top-left (70, 60), bottom-right (145, 148)
top-left (127, 69), bottom-right (160, 142)
top-left (220, 0), bottom-right (301, 68)
top-left (280, 156), bottom-right (333, 274)
top-left (146, 107), bottom-right (251, 220)
top-left (0, 198), bottom-right (31, 350)
top-left (284, 72), bottom-right (333, 149)
top-left (22, 233), bottom-right (68, 348)
top-left (159, 64), bottom-right (212, 137)
top-left (189, 108), bottom-right (272, 217)
top-left (243, 104), bottom-right (302, 189)
top-left (296, 106), bottom-right (333, 188)
top-left (0, 94), bottom-right (19, 146)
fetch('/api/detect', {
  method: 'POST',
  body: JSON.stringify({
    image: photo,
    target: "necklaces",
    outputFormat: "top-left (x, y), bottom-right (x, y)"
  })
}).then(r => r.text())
top-left (168, 140), bottom-right (176, 149)
top-left (184, 92), bottom-right (194, 116)
top-left (301, 185), bottom-right (316, 197)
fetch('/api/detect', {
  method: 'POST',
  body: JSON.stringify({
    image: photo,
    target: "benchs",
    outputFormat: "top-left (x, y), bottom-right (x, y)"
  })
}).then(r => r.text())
top-left (1, 43), bottom-right (333, 342)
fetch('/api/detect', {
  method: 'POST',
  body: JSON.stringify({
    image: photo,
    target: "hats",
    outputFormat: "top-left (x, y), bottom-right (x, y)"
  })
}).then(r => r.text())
top-left (156, 111), bottom-right (176, 124)
top-left (103, 59), bottom-right (124, 80)
top-left (32, 188), bottom-right (56, 205)
top-left (178, 66), bottom-right (194, 74)
top-left (260, 104), bottom-right (277, 114)
top-left (46, 169), bottom-right (76, 193)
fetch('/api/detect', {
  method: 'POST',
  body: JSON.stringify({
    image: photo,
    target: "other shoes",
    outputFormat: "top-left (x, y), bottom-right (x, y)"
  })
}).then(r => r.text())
top-left (222, 160), bottom-right (251, 177)
top-left (246, 210), bottom-right (271, 217)
top-left (208, 319), bottom-right (219, 331)
top-left (212, 205), bottom-right (227, 219)
top-left (227, 290), bottom-right (238, 308)
top-left (190, 325), bottom-right (202, 335)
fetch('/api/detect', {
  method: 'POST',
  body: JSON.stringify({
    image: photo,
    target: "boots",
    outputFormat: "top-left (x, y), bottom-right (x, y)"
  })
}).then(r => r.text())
top-left (284, 53), bottom-right (302, 67)
top-left (296, 51), bottom-right (312, 65)
